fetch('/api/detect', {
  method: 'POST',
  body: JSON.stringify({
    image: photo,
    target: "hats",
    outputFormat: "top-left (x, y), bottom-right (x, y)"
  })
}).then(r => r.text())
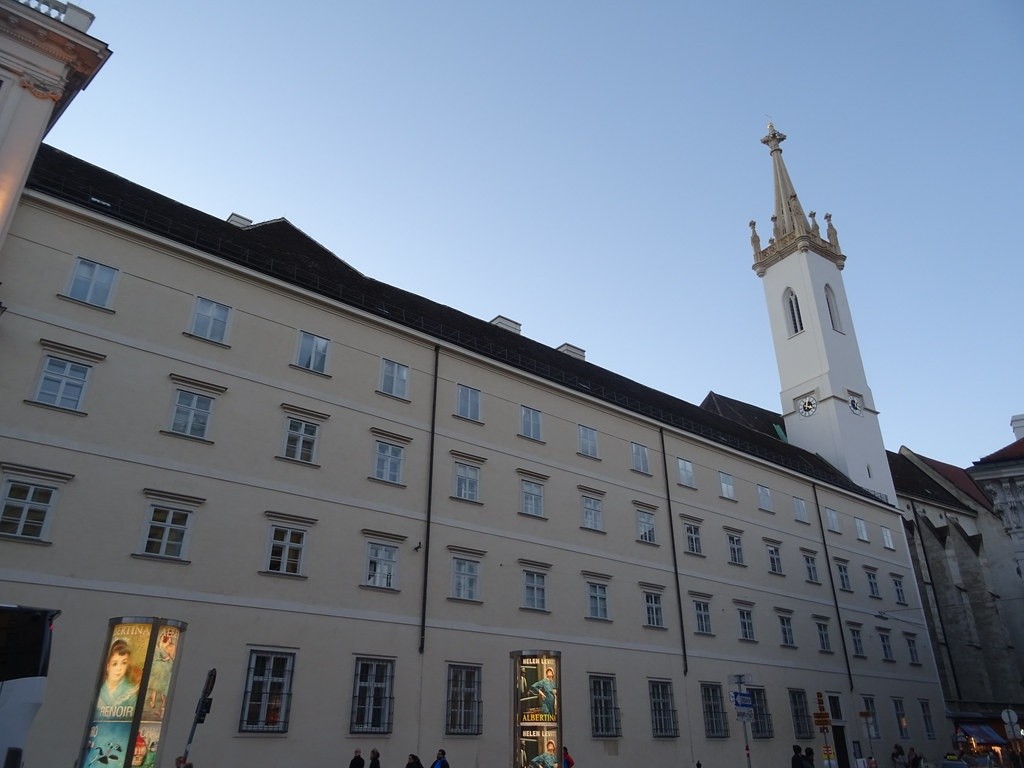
top-left (805, 747), bottom-right (813, 755)
top-left (793, 745), bottom-right (802, 754)
top-left (547, 740), bottom-right (556, 748)
top-left (520, 667), bottom-right (526, 673)
top-left (546, 667), bottom-right (554, 676)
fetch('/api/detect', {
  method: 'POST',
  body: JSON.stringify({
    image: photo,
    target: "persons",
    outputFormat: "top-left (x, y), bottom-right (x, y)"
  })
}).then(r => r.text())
top-left (430, 749), bottom-right (449, 768)
top-left (791, 744), bottom-right (805, 768)
top-left (891, 743), bottom-right (905, 768)
top-left (531, 667), bottom-right (557, 715)
top-left (369, 749), bottom-right (380, 768)
top-left (520, 666), bottom-right (529, 721)
top-left (563, 747), bottom-right (575, 768)
top-left (1009, 748), bottom-right (1020, 768)
top-left (908, 747), bottom-right (917, 768)
top-left (531, 740), bottom-right (558, 768)
top-left (92, 639), bottom-right (137, 722)
top-left (516, 741), bottom-right (529, 768)
top-left (405, 753), bottom-right (424, 768)
top-left (349, 748), bottom-right (364, 768)
top-left (802, 747), bottom-right (814, 768)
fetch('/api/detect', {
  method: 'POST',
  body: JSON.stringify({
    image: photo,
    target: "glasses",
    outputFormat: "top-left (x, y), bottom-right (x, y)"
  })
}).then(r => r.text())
top-left (355, 751), bottom-right (359, 752)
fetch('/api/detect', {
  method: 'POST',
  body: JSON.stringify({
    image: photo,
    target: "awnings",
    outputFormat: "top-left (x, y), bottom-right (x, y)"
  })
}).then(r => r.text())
top-left (958, 721), bottom-right (1006, 746)
